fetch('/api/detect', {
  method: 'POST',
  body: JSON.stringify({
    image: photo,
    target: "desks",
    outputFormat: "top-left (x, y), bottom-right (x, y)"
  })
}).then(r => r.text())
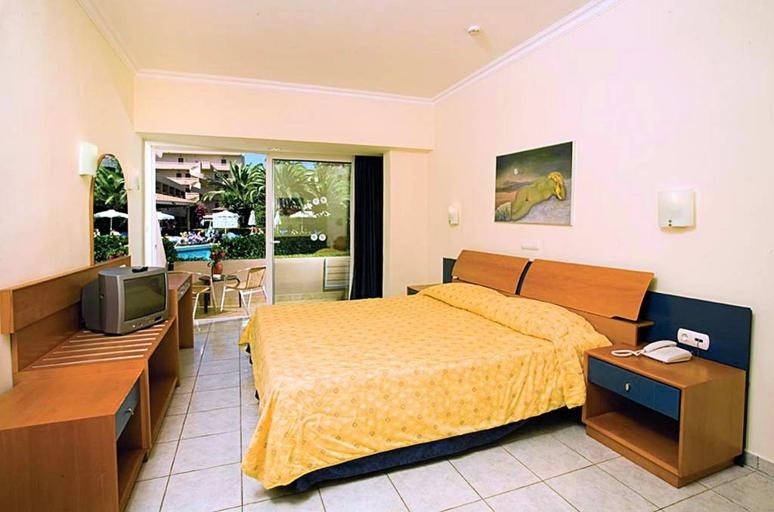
top-left (166, 271), bottom-right (194, 347)
top-left (200, 275), bottom-right (242, 313)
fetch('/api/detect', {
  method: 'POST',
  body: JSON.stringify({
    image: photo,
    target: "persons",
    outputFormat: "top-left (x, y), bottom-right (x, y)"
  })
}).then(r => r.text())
top-left (174, 226), bottom-right (220, 246)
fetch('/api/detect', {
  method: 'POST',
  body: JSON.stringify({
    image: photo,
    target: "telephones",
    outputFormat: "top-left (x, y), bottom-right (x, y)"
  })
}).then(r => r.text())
top-left (640, 340), bottom-right (692, 364)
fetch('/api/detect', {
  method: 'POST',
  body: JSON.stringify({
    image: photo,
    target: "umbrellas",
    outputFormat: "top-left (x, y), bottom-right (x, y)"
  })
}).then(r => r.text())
top-left (290, 211), bottom-right (313, 232)
top-left (203, 210), bottom-right (240, 233)
top-left (248, 210), bottom-right (257, 234)
top-left (157, 212), bottom-right (175, 220)
top-left (274, 210), bottom-right (282, 235)
top-left (94, 209), bottom-right (128, 229)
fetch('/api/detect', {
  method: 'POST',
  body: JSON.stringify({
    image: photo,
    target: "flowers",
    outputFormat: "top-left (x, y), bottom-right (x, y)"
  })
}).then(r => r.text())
top-left (206, 251), bottom-right (225, 267)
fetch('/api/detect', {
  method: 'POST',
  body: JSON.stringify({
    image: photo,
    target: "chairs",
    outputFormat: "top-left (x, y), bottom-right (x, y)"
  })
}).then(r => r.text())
top-left (220, 266), bottom-right (269, 316)
top-left (192, 273), bottom-right (218, 318)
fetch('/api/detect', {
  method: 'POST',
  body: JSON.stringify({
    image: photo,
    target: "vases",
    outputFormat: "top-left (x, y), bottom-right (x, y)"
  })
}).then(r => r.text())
top-left (211, 262), bottom-right (223, 277)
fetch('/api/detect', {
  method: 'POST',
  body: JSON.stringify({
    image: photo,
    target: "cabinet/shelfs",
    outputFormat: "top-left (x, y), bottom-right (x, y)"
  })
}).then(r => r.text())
top-left (0, 286), bottom-right (181, 512)
top-left (407, 282), bottom-right (441, 295)
top-left (581, 342), bottom-right (746, 489)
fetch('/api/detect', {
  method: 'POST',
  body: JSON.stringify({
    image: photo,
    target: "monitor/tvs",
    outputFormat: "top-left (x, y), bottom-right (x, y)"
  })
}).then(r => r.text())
top-left (81, 266), bottom-right (168, 335)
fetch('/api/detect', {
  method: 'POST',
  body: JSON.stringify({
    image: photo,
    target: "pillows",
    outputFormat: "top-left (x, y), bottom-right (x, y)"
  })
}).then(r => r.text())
top-left (416, 283), bottom-right (502, 317)
top-left (468, 297), bottom-right (605, 409)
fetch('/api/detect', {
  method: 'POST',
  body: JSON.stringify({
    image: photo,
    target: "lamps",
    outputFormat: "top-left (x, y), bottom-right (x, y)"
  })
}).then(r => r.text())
top-left (78, 142), bottom-right (97, 177)
top-left (657, 188), bottom-right (695, 228)
top-left (448, 206), bottom-right (459, 225)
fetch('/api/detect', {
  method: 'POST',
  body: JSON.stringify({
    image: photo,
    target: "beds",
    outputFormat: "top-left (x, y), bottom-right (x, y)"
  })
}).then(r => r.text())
top-left (236, 249), bottom-right (654, 494)
